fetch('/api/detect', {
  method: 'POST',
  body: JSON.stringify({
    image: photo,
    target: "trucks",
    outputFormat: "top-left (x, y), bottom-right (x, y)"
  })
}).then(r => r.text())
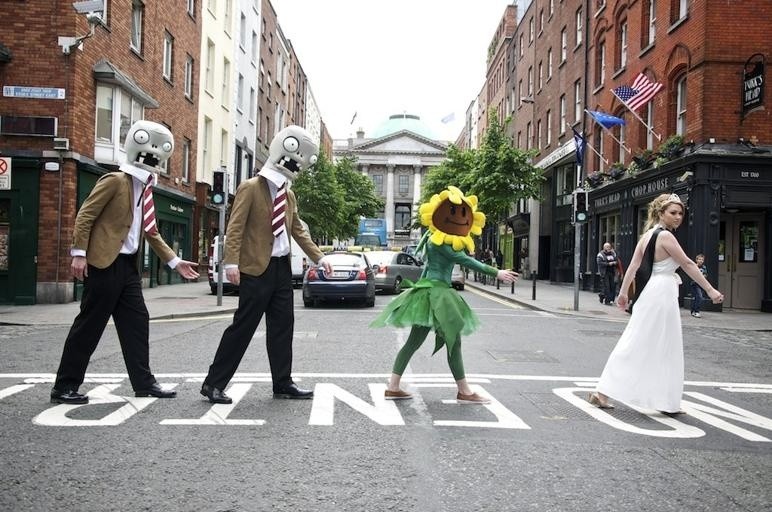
top-left (290, 218), bottom-right (310, 281)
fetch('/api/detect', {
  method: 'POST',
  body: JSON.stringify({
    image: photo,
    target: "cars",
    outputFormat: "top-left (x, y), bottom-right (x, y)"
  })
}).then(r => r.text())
top-left (299, 244), bottom-right (467, 309)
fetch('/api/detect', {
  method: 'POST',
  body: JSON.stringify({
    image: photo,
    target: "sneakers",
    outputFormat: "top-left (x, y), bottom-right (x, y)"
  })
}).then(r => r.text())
top-left (456, 392), bottom-right (491, 405)
top-left (384, 388), bottom-right (413, 402)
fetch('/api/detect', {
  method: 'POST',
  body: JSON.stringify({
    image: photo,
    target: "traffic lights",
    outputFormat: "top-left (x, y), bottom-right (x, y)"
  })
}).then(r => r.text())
top-left (574, 191), bottom-right (591, 225)
top-left (211, 170), bottom-right (227, 205)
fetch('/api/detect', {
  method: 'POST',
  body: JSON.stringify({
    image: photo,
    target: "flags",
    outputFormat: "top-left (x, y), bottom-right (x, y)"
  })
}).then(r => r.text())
top-left (591, 112), bottom-right (625, 130)
top-left (574, 128), bottom-right (586, 167)
top-left (614, 71), bottom-right (663, 112)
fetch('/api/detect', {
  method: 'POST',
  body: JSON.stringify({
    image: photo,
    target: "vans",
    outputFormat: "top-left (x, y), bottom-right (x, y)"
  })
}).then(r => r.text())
top-left (203, 234), bottom-right (240, 299)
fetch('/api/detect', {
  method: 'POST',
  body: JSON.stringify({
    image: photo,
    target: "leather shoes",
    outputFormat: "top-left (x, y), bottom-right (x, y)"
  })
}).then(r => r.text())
top-left (50, 386), bottom-right (89, 405)
top-left (273, 382), bottom-right (313, 399)
top-left (135, 382), bottom-right (178, 398)
top-left (199, 382), bottom-right (233, 404)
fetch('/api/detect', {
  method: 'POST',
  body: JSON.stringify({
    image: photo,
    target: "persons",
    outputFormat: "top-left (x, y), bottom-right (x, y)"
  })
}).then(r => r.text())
top-left (690, 252), bottom-right (708, 319)
top-left (368, 185), bottom-right (519, 404)
top-left (589, 192), bottom-right (725, 414)
top-left (200, 126), bottom-right (333, 403)
top-left (477, 246), bottom-right (504, 285)
top-left (612, 250), bottom-right (624, 303)
top-left (51, 119), bottom-right (200, 404)
top-left (597, 242), bottom-right (618, 306)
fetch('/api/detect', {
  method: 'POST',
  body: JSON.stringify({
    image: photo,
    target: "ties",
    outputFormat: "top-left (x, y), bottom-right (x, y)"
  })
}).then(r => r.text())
top-left (271, 183), bottom-right (287, 238)
top-left (142, 176), bottom-right (157, 238)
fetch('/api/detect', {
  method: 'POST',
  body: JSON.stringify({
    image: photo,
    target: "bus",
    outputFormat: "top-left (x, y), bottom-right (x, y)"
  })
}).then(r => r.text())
top-left (338, 217), bottom-right (389, 248)
top-left (339, 231), bottom-right (383, 249)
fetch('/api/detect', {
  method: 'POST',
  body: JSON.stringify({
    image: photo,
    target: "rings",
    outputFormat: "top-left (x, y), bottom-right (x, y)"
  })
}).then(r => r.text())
top-left (719, 298), bottom-right (722, 301)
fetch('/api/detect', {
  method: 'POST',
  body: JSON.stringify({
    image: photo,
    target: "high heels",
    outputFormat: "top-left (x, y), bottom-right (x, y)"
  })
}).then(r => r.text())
top-left (660, 408), bottom-right (686, 417)
top-left (588, 393), bottom-right (615, 410)
